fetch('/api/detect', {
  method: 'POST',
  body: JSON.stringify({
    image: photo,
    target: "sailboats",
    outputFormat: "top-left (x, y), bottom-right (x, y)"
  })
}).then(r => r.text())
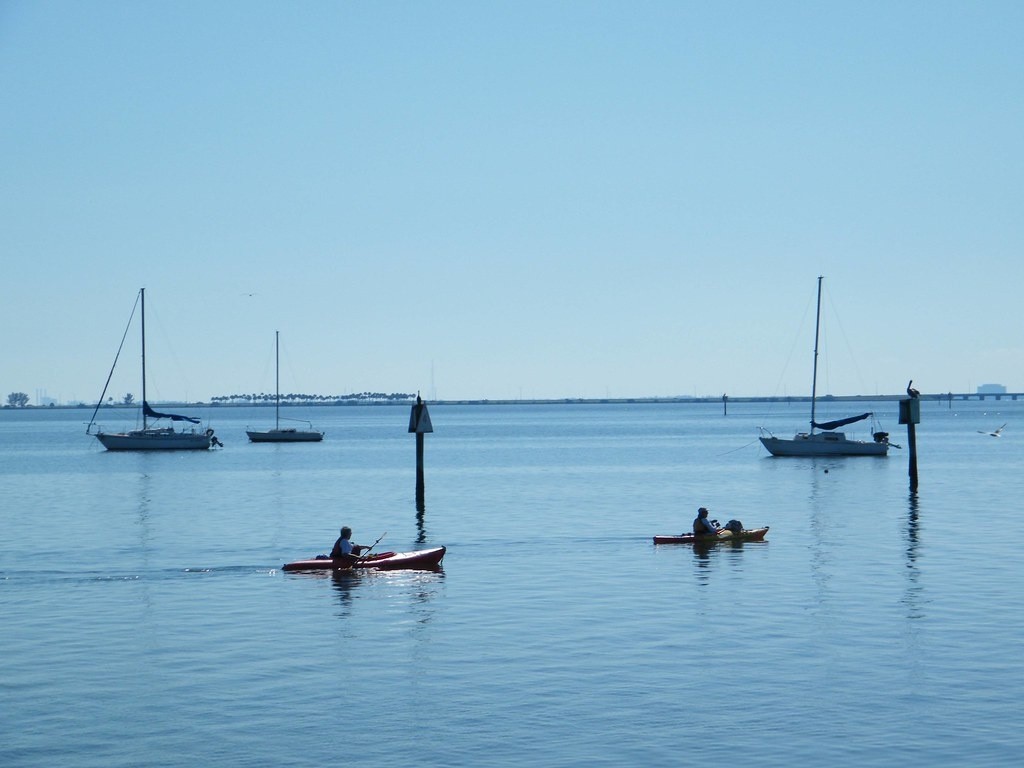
top-left (82, 286), bottom-right (226, 450)
top-left (245, 330), bottom-right (328, 441)
top-left (759, 274), bottom-right (905, 456)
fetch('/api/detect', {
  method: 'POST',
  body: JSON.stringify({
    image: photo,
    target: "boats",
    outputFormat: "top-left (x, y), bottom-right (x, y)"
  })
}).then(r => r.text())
top-left (281, 544), bottom-right (448, 571)
top-left (652, 526), bottom-right (770, 543)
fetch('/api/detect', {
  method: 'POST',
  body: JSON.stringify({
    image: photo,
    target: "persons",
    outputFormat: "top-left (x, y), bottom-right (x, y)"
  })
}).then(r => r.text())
top-left (330, 526), bottom-right (373, 560)
top-left (693, 508), bottom-right (720, 535)
top-left (874, 432), bottom-right (889, 443)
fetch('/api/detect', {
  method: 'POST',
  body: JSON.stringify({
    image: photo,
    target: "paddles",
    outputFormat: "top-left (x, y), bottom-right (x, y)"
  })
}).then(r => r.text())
top-left (337, 533), bottom-right (386, 570)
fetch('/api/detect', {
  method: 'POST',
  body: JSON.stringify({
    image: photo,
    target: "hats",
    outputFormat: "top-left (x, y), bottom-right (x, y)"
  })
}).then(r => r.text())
top-left (341, 527), bottom-right (351, 537)
top-left (698, 508), bottom-right (710, 513)
